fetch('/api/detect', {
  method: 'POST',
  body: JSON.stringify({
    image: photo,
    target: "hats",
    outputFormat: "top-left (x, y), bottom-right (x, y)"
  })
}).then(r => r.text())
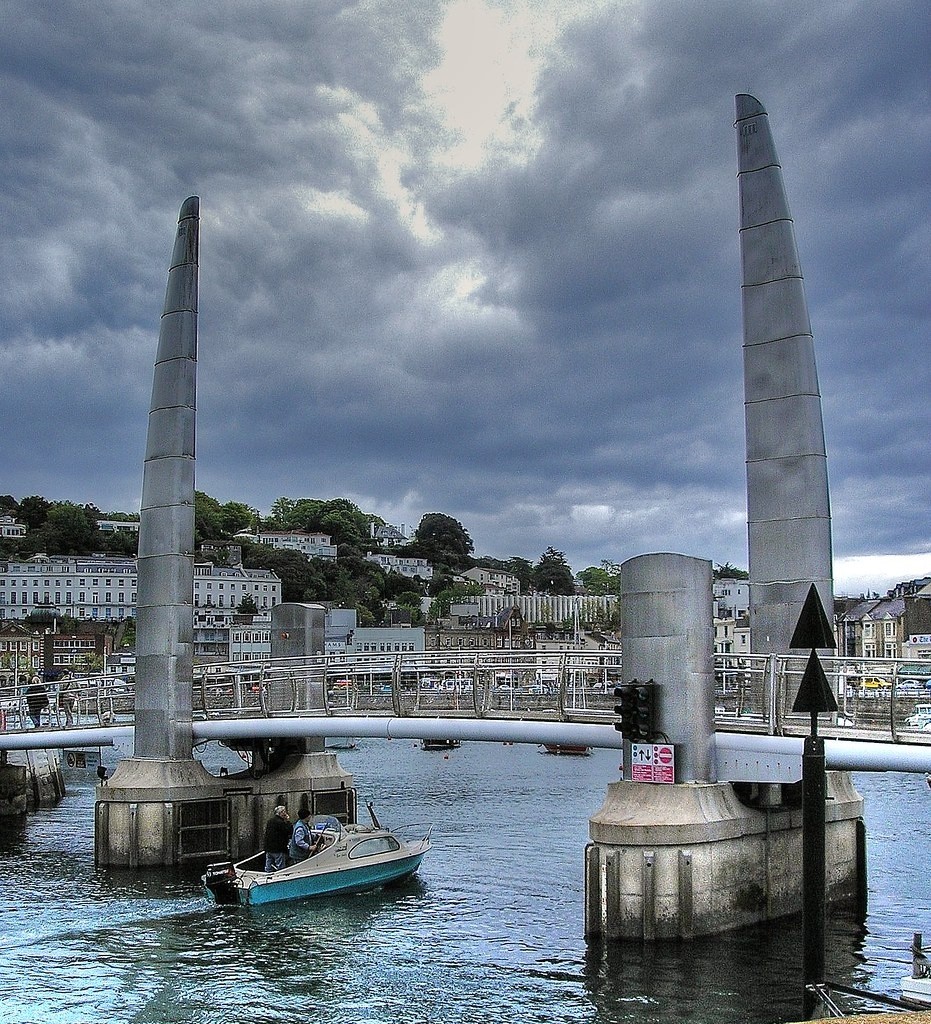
top-left (298, 809), bottom-right (311, 819)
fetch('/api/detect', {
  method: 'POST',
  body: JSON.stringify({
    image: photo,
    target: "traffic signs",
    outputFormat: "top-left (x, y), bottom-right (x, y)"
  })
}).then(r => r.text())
top-left (631, 743), bottom-right (675, 784)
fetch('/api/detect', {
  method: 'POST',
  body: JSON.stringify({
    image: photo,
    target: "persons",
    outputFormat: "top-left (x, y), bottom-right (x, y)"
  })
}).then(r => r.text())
top-left (26, 677), bottom-right (49, 728)
top-left (263, 806), bottom-right (319, 872)
top-left (59, 669), bottom-right (81, 725)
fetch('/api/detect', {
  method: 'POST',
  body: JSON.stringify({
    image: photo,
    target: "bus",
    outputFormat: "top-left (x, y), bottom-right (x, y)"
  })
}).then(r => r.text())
top-left (420, 677), bottom-right (474, 691)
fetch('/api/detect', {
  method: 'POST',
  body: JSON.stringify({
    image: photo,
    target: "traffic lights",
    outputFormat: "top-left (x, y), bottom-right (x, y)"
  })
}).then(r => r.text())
top-left (613, 678), bottom-right (656, 744)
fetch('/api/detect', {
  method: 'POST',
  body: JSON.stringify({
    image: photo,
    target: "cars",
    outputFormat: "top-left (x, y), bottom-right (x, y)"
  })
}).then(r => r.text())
top-left (498, 685), bottom-right (515, 691)
top-left (896, 680), bottom-right (923, 689)
top-left (333, 684), bottom-right (353, 690)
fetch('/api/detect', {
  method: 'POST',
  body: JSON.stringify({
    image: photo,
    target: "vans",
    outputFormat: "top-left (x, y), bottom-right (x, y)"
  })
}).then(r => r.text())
top-left (905, 704), bottom-right (931, 726)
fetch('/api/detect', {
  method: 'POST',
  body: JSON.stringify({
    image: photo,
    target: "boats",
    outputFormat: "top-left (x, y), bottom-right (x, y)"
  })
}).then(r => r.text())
top-left (201, 801), bottom-right (434, 906)
top-left (325, 736), bottom-right (363, 749)
top-left (420, 738), bottom-right (462, 752)
top-left (543, 742), bottom-right (593, 756)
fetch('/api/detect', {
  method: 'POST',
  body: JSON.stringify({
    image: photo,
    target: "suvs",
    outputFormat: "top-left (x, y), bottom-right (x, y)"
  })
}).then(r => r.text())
top-left (860, 677), bottom-right (892, 689)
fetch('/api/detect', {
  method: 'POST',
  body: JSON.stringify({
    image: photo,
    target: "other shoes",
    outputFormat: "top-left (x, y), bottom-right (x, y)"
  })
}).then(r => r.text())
top-left (65, 722), bottom-right (73, 726)
top-left (34, 723), bottom-right (40, 727)
